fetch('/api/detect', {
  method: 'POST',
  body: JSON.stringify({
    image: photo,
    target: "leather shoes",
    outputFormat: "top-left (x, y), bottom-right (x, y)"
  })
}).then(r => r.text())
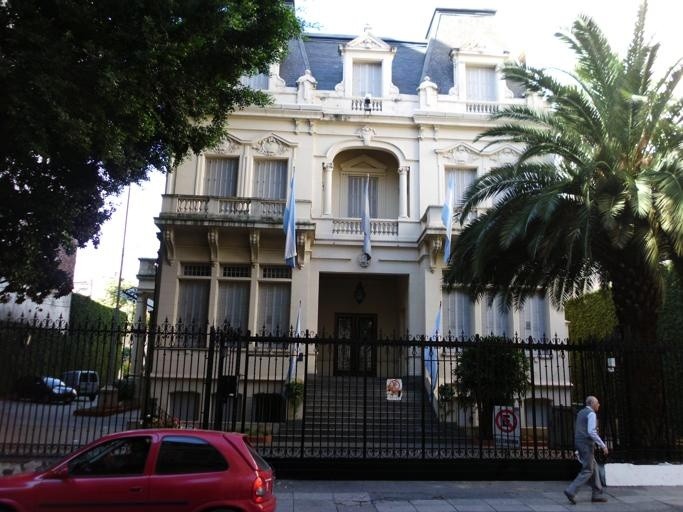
top-left (563, 490), bottom-right (576, 504)
top-left (591, 496), bottom-right (608, 502)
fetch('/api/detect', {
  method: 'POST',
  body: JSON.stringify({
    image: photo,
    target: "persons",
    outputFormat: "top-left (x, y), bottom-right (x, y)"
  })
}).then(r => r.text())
top-left (561, 394), bottom-right (610, 504)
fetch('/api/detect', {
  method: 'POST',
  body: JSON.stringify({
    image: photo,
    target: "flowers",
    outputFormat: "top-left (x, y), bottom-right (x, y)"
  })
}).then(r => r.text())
top-left (285, 379), bottom-right (304, 402)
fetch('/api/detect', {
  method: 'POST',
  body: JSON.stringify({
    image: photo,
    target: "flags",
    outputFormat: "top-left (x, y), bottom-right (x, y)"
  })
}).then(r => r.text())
top-left (282, 172), bottom-right (298, 269)
top-left (441, 174), bottom-right (453, 265)
top-left (279, 307), bottom-right (302, 400)
top-left (359, 178), bottom-right (372, 261)
top-left (423, 304), bottom-right (444, 391)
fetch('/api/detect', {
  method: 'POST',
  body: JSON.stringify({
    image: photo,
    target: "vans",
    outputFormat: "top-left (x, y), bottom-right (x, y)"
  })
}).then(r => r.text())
top-left (60, 370), bottom-right (100, 402)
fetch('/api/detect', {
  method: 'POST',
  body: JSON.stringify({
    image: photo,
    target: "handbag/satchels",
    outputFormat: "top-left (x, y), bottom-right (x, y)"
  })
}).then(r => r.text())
top-left (594, 447), bottom-right (608, 464)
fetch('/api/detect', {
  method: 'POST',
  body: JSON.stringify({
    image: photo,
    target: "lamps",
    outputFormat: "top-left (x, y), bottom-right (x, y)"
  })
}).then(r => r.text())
top-left (607, 351), bottom-right (616, 374)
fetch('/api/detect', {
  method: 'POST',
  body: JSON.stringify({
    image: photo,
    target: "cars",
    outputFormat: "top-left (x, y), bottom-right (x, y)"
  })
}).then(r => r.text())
top-left (10, 375), bottom-right (78, 404)
top-left (2, 427), bottom-right (277, 511)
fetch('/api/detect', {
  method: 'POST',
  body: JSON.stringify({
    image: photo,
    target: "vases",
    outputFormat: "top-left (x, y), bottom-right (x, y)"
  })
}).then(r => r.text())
top-left (290, 399), bottom-right (299, 408)
